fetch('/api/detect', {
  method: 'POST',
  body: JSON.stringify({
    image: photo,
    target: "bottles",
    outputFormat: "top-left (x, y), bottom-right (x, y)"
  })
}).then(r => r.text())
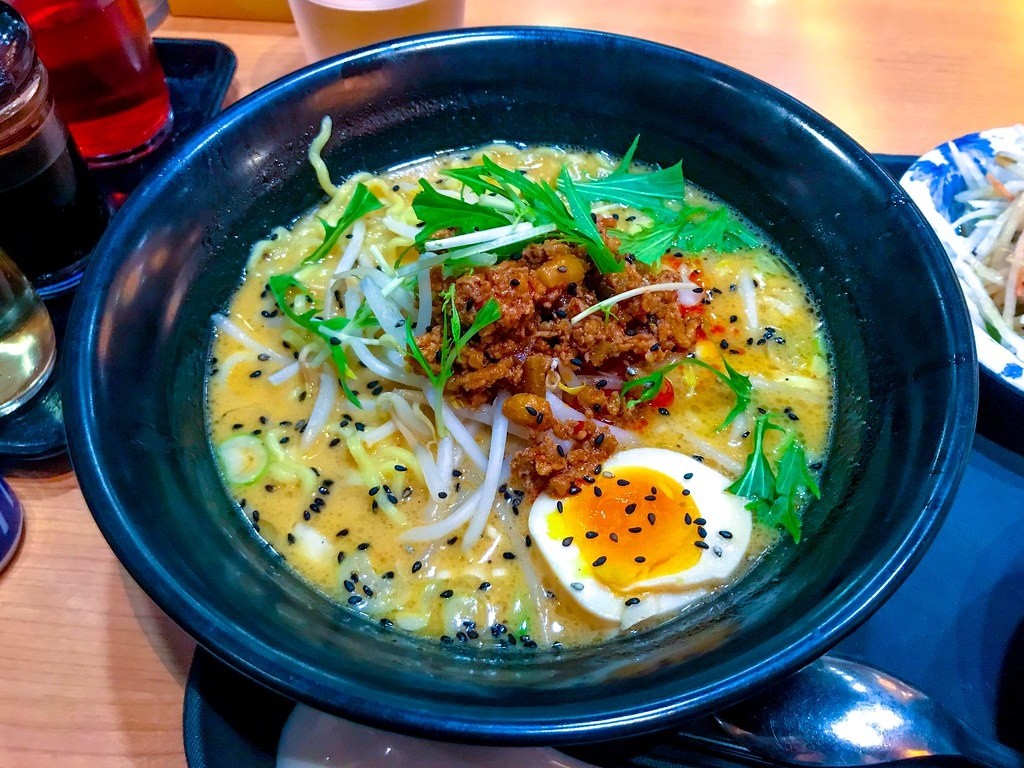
top-left (0, 2), bottom-right (118, 301)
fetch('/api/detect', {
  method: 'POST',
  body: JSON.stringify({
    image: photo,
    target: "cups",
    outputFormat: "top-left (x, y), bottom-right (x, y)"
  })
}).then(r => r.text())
top-left (286, 0), bottom-right (466, 64)
top-left (0, 248), bottom-right (57, 417)
top-left (1, 0), bottom-right (175, 168)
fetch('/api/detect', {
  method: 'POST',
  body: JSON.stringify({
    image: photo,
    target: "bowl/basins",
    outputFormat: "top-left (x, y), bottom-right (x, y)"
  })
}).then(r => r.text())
top-left (61, 26), bottom-right (981, 748)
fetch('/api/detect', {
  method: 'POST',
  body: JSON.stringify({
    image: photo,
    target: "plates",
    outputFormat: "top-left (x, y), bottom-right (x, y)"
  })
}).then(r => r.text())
top-left (898, 127), bottom-right (1024, 395)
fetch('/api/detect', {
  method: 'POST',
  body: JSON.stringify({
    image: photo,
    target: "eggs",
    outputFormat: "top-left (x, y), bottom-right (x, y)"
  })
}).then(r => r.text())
top-left (526, 446), bottom-right (754, 622)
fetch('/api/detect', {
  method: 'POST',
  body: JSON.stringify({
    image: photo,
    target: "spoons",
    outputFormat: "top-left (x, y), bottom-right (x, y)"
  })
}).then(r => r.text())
top-left (717, 652), bottom-right (1024, 768)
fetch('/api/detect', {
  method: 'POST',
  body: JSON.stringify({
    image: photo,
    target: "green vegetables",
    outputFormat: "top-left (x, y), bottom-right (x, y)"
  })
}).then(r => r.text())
top-left (268, 132), bottom-right (824, 547)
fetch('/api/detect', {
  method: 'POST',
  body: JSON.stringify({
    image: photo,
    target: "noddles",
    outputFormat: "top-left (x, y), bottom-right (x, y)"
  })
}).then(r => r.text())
top-left (209, 115), bottom-right (838, 651)
